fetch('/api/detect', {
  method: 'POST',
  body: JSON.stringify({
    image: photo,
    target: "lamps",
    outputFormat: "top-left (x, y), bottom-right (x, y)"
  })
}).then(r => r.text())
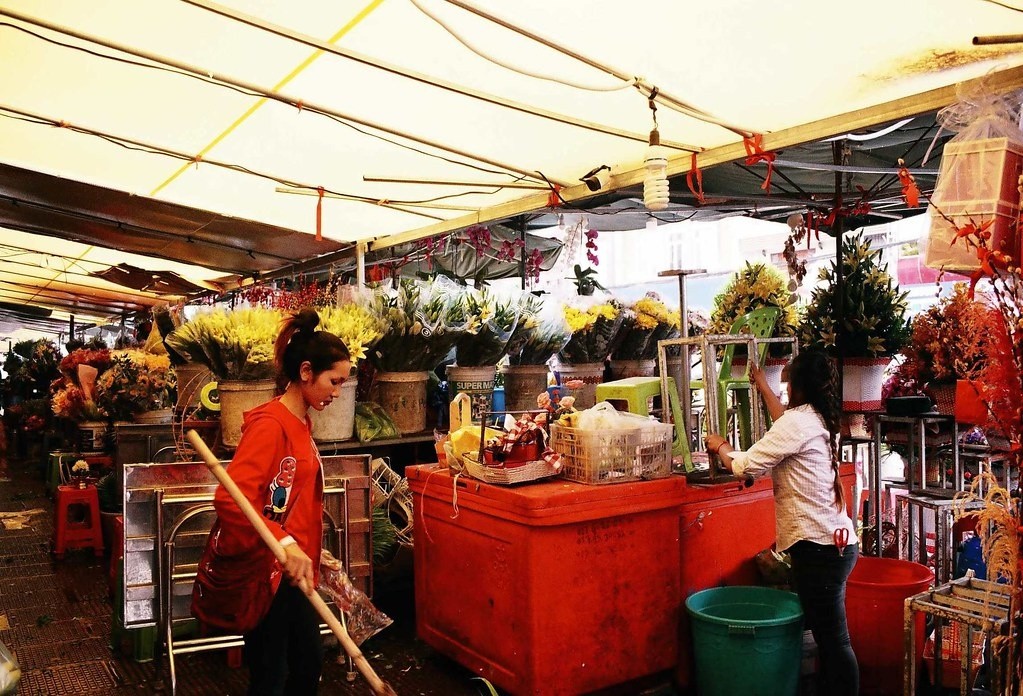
top-left (640, 90), bottom-right (674, 212)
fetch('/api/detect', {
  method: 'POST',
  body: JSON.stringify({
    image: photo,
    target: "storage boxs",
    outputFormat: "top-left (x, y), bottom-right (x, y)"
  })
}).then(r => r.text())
top-left (925, 136), bottom-right (1023, 280)
top-left (549, 421), bottom-right (675, 484)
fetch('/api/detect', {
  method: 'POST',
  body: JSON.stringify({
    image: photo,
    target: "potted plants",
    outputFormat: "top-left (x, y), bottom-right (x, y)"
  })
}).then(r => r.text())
top-left (929, 379), bottom-right (962, 416)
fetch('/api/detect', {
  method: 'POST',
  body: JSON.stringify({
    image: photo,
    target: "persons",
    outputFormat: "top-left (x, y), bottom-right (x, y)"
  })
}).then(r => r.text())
top-left (703, 349), bottom-right (860, 696)
top-left (210, 306), bottom-right (352, 696)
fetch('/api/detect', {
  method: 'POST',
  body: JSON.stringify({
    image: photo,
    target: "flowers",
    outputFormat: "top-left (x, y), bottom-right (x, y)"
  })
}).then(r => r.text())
top-left (710, 261), bottom-right (799, 358)
top-left (171, 286), bottom-right (686, 376)
top-left (797, 238), bottom-right (913, 357)
top-left (903, 282), bottom-right (1007, 392)
top-left (72, 460), bottom-right (91, 479)
top-left (2, 338), bottom-right (169, 454)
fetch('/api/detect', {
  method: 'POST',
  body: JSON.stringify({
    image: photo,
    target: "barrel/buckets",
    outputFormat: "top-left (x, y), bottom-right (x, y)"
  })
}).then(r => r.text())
top-left (685, 585), bottom-right (806, 696)
top-left (814, 557), bottom-right (935, 696)
top-left (611, 361), bottom-right (657, 414)
top-left (175, 363), bottom-right (215, 411)
top-left (446, 364), bottom-right (498, 428)
top-left (657, 357), bottom-right (691, 410)
top-left (503, 364), bottom-right (552, 420)
top-left (955, 537), bottom-right (1013, 620)
top-left (559, 362), bottom-right (607, 412)
top-left (374, 369), bottom-right (430, 435)
top-left (217, 376), bottom-right (279, 450)
top-left (306, 375), bottom-right (358, 444)
top-left (483, 443), bottom-right (542, 471)
top-left (77, 421), bottom-right (109, 456)
top-left (134, 407), bottom-right (173, 425)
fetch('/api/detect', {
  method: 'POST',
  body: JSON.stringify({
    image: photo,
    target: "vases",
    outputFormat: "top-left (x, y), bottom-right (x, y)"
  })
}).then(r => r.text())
top-left (376, 369), bottom-right (432, 439)
top-left (552, 361), bottom-right (605, 417)
top-left (611, 356), bottom-right (662, 416)
top-left (74, 474), bottom-right (88, 491)
top-left (662, 356), bottom-right (698, 408)
top-left (831, 355), bottom-right (889, 413)
top-left (175, 362), bottom-right (217, 414)
top-left (722, 357), bottom-right (791, 406)
top-left (443, 360), bottom-right (496, 431)
top-left (304, 373), bottom-right (355, 446)
top-left (501, 364), bottom-right (552, 425)
top-left (216, 377), bottom-right (281, 448)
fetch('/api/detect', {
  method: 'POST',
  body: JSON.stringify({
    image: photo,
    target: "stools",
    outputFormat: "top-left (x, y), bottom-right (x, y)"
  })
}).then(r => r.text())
top-left (53, 477), bottom-right (106, 560)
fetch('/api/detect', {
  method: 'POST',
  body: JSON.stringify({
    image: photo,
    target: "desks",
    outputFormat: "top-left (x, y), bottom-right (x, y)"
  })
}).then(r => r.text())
top-left (119, 453), bottom-right (382, 696)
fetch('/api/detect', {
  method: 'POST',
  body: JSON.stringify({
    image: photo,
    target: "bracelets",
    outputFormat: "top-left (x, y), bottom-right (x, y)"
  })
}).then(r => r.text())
top-left (716, 441), bottom-right (727, 454)
